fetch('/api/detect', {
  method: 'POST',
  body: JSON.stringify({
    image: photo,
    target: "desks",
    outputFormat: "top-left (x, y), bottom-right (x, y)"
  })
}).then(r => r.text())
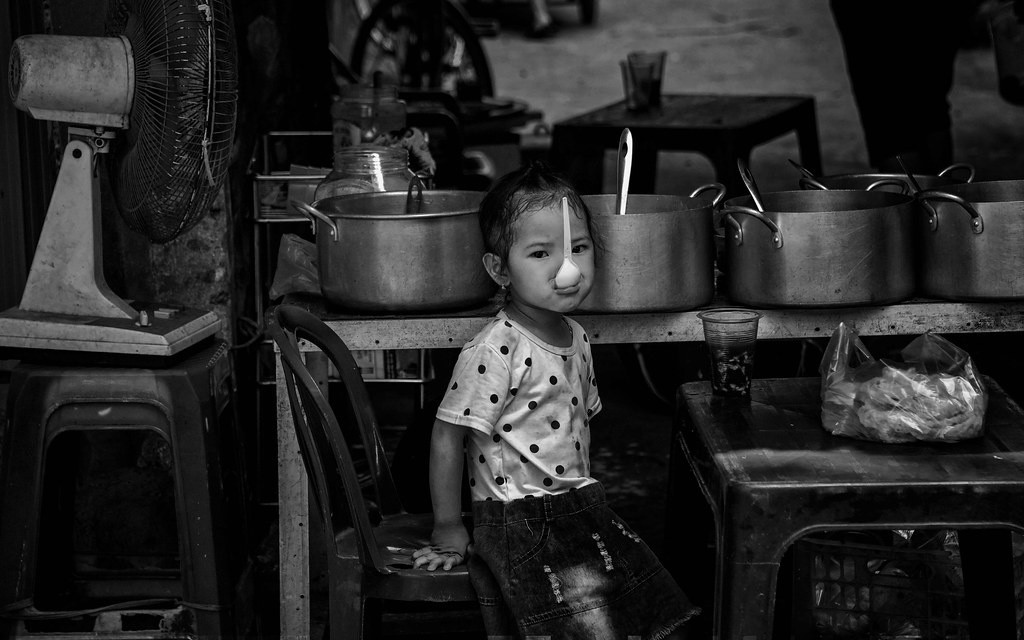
top-left (547, 90), bottom-right (823, 207)
top-left (666, 361), bottom-right (1023, 640)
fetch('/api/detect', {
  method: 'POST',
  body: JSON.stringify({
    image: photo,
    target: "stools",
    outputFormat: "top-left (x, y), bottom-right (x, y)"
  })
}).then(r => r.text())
top-left (1, 334), bottom-right (240, 639)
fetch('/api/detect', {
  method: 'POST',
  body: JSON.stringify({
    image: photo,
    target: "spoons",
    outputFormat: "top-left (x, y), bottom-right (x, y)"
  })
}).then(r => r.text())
top-left (555, 196), bottom-right (581, 289)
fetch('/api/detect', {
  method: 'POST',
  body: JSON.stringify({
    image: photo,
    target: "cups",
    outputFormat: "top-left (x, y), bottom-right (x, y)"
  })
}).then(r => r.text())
top-left (696, 308), bottom-right (762, 409)
top-left (619, 51), bottom-right (666, 120)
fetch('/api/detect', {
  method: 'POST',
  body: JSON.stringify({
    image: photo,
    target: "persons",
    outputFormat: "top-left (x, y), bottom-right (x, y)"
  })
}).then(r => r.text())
top-left (411, 167), bottom-right (704, 640)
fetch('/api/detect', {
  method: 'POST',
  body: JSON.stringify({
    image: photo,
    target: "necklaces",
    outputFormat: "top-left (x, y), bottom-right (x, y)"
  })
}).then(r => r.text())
top-left (504, 306), bottom-right (573, 347)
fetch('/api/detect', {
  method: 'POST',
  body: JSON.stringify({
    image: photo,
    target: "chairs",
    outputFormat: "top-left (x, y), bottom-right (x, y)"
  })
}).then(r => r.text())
top-left (266, 301), bottom-right (484, 640)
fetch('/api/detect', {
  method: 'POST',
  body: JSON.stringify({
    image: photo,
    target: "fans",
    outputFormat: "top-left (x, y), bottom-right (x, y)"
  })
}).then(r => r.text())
top-left (0, 0), bottom-right (240, 366)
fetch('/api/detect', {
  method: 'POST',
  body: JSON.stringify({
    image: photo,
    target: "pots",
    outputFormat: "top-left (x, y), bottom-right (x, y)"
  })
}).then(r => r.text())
top-left (290, 155), bottom-right (1024, 312)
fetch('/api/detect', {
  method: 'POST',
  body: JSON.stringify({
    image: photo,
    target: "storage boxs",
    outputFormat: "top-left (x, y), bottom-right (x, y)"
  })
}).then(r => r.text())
top-left (796, 529), bottom-right (972, 640)
